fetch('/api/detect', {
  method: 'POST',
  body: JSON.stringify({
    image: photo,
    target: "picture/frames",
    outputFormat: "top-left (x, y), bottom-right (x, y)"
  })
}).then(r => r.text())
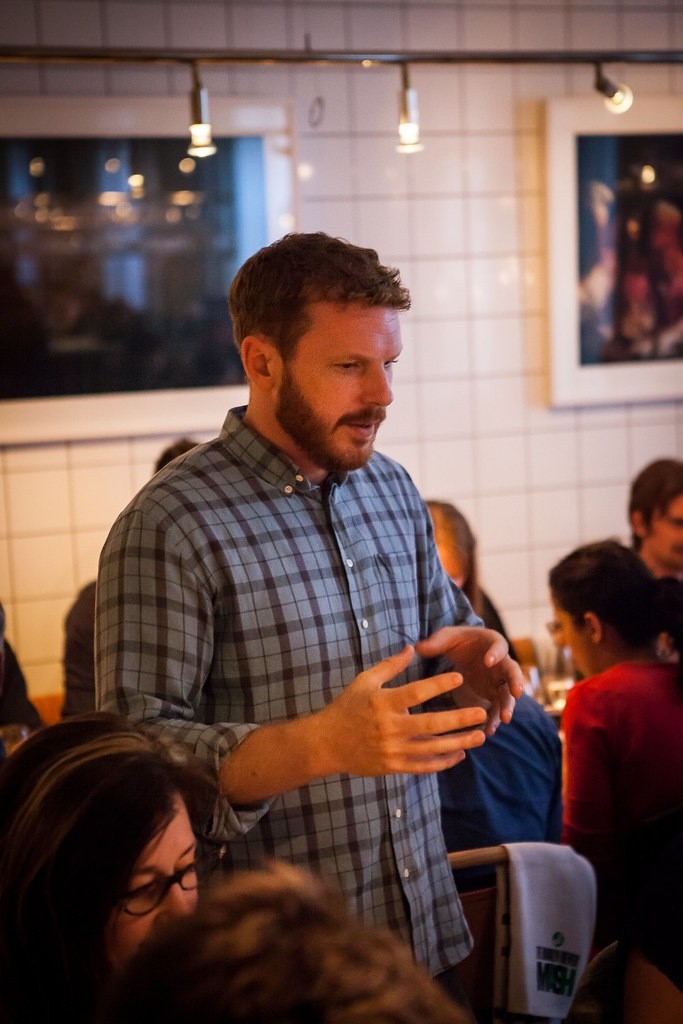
top-left (541, 90), bottom-right (683, 409)
top-left (0, 92), bottom-right (301, 454)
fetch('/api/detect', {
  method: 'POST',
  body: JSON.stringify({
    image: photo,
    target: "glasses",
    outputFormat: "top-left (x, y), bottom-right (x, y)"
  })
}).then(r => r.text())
top-left (118, 863), bottom-right (198, 917)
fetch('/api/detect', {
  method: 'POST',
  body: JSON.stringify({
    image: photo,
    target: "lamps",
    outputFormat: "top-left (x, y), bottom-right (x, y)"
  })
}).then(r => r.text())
top-left (186, 59), bottom-right (217, 158)
top-left (392, 57), bottom-right (425, 155)
top-left (592, 58), bottom-right (634, 116)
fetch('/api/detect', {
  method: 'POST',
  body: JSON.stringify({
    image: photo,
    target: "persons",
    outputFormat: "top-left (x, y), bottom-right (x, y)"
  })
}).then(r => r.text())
top-left (60, 440), bottom-right (204, 722)
top-left (0, 602), bottom-right (45, 742)
top-left (101, 863), bottom-right (472, 1024)
top-left (550, 540), bottom-right (683, 1001)
top-left (425, 499), bottom-right (518, 663)
top-left (0, 715), bottom-right (202, 1024)
top-left (93, 231), bottom-right (523, 1004)
top-left (437, 691), bottom-right (562, 889)
top-left (626, 461), bottom-right (683, 660)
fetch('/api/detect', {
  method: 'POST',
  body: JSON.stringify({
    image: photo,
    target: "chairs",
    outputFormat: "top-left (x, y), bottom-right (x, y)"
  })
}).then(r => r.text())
top-left (440, 842), bottom-right (598, 1024)
